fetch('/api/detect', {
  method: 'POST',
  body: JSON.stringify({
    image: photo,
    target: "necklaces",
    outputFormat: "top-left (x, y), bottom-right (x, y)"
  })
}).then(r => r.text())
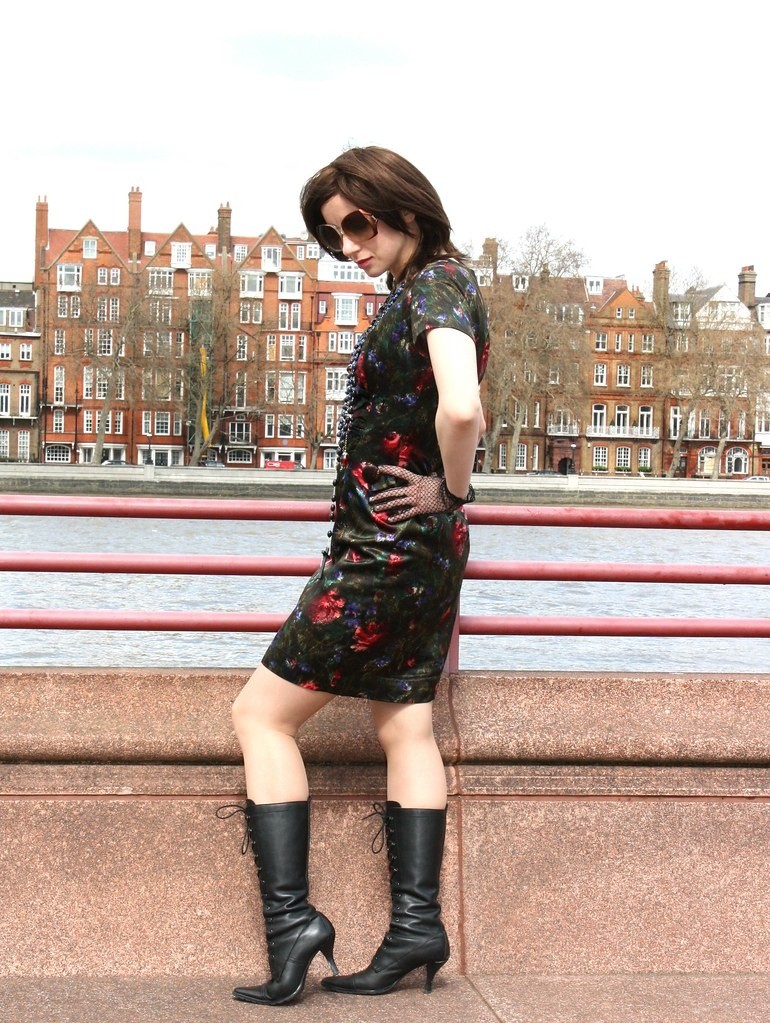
top-left (317, 282), bottom-right (405, 580)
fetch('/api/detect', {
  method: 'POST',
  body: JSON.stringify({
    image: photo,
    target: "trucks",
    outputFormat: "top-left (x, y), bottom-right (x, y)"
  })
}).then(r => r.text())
top-left (264, 460), bottom-right (305, 470)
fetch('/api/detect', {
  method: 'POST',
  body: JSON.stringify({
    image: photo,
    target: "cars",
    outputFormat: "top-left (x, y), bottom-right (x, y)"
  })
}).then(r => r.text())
top-left (741, 476), bottom-right (770, 481)
top-left (102, 460), bottom-right (130, 466)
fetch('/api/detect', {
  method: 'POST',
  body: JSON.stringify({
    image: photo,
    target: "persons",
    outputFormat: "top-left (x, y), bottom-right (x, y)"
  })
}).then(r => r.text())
top-left (216, 146), bottom-right (491, 1005)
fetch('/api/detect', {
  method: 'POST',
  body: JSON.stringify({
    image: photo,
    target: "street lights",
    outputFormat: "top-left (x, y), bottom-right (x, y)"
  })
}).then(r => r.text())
top-left (146, 433), bottom-right (155, 465)
top-left (569, 443), bottom-right (578, 475)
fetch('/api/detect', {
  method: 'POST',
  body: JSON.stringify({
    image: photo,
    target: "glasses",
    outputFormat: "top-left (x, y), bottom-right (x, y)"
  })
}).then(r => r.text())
top-left (317, 209), bottom-right (378, 254)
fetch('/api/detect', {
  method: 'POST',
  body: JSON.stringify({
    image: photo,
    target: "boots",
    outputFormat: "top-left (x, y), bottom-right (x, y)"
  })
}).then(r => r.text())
top-left (216, 799), bottom-right (340, 1007)
top-left (321, 799), bottom-right (449, 995)
